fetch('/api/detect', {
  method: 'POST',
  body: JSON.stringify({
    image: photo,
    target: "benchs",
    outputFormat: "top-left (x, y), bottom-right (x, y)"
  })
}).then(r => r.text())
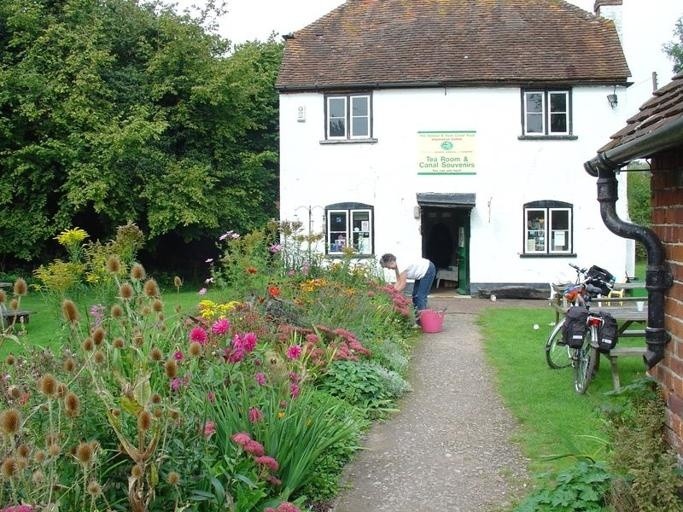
top-left (552, 280), bottom-right (647, 392)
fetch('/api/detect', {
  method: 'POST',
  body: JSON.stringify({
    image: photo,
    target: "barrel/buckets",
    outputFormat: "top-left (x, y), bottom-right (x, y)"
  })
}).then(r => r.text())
top-left (419, 309), bottom-right (444, 334)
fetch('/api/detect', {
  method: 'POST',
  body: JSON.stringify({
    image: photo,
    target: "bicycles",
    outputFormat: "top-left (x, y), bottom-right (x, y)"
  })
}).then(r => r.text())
top-left (545, 262), bottom-right (619, 395)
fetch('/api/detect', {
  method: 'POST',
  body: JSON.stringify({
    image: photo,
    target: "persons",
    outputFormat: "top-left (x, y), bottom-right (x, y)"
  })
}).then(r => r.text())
top-left (378, 253), bottom-right (436, 329)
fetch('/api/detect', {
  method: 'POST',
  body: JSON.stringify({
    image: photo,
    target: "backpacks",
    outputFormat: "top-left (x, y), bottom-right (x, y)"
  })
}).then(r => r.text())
top-left (597, 311), bottom-right (618, 352)
top-left (584, 265), bottom-right (616, 296)
top-left (563, 305), bottom-right (588, 348)
